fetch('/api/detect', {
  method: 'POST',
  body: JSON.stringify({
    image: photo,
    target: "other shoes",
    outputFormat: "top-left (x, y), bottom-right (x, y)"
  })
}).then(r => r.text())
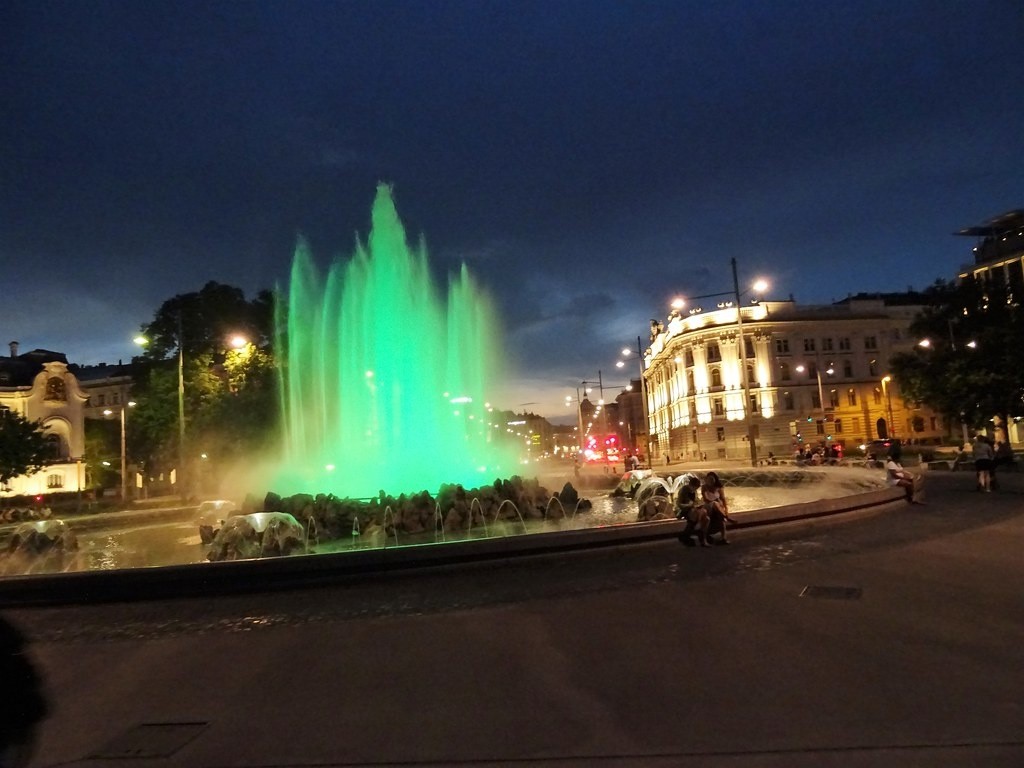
top-left (986, 489), bottom-right (992, 493)
top-left (720, 537), bottom-right (730, 545)
top-left (730, 520), bottom-right (738, 524)
top-left (700, 541), bottom-right (712, 547)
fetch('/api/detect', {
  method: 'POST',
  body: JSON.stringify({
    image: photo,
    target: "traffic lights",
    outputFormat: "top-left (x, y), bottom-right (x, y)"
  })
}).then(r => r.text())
top-left (796, 431), bottom-right (803, 443)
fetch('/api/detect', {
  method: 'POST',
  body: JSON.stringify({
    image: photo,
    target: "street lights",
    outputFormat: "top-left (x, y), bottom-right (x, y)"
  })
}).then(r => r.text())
top-left (103, 377), bottom-right (137, 503)
top-left (795, 365), bottom-right (834, 446)
top-left (132, 332), bottom-right (248, 505)
top-left (581, 370), bottom-right (607, 434)
top-left (616, 336), bottom-right (652, 469)
top-left (881, 375), bottom-right (892, 438)
top-left (669, 256), bottom-right (773, 469)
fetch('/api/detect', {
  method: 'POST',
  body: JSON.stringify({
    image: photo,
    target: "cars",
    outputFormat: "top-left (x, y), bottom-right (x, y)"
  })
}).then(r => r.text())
top-left (870, 437), bottom-right (901, 460)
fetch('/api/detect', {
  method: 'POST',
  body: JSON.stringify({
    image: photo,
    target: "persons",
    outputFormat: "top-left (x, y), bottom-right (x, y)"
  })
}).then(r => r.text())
top-left (791, 434), bottom-right (903, 466)
top-left (89, 490), bottom-right (96, 501)
top-left (674, 476), bottom-right (709, 548)
top-left (613, 454), bottom-right (639, 474)
top-left (951, 446), bottom-right (968, 471)
top-left (701, 472), bottom-right (738, 544)
top-left (972, 435), bottom-right (997, 493)
top-left (574, 458), bottom-right (581, 478)
top-left (991, 441), bottom-right (1014, 467)
top-left (703, 452), bottom-right (706, 461)
top-left (767, 452), bottom-right (775, 466)
top-left (887, 453), bottom-right (915, 505)
